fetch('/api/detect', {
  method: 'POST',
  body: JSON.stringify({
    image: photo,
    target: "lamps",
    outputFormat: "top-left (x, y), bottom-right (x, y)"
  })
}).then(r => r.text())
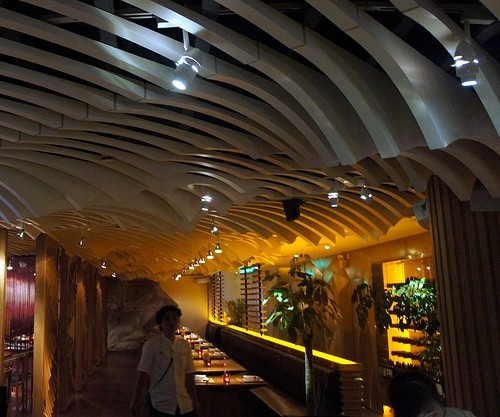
top-left (327, 192), bottom-right (339, 208)
top-left (171, 29), bottom-right (203, 90)
top-left (358, 178), bottom-right (373, 206)
top-left (282, 198), bottom-right (302, 222)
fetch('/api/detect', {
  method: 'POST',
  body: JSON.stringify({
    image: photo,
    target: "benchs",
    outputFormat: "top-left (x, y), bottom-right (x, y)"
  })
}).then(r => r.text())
top-left (250, 384), bottom-right (307, 417)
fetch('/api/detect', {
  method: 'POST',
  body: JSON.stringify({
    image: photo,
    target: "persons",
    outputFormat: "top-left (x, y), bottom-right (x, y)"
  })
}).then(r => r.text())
top-left (389, 367), bottom-right (476, 417)
top-left (130, 305), bottom-right (200, 417)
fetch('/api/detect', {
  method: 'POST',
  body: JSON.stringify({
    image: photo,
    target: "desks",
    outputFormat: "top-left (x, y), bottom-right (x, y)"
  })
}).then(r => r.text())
top-left (193, 358), bottom-right (248, 374)
top-left (194, 374), bottom-right (268, 417)
top-left (191, 348), bottom-right (228, 359)
top-left (184, 332), bottom-right (215, 348)
